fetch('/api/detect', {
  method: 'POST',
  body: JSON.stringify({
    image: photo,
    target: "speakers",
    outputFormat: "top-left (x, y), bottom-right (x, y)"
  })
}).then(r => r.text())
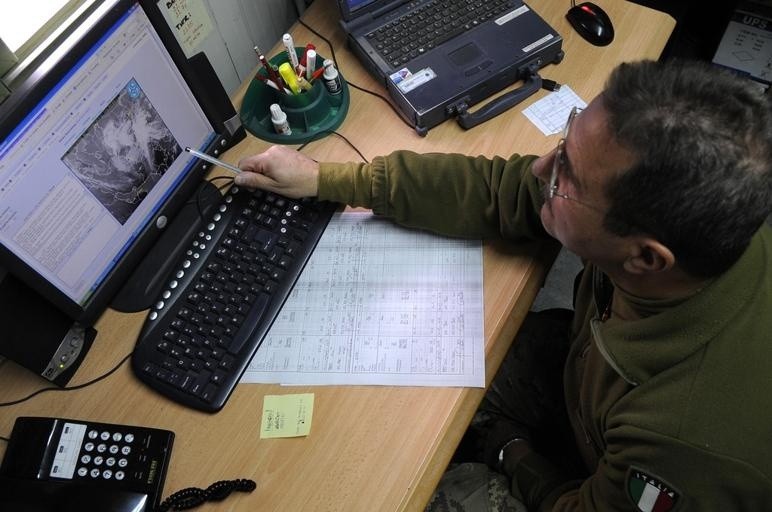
top-left (188, 51), bottom-right (247, 154)
top-left (0, 242), bottom-right (98, 388)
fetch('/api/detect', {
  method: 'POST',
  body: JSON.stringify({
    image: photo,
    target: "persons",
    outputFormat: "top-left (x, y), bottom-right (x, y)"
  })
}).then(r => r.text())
top-left (232, 56), bottom-right (771, 512)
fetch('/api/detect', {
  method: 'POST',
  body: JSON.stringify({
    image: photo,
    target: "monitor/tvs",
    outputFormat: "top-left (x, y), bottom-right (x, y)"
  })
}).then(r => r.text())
top-left (0, 0), bottom-right (232, 330)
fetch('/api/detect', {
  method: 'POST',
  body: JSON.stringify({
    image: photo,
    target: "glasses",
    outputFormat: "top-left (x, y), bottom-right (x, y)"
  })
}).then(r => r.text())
top-left (549, 107), bottom-right (610, 214)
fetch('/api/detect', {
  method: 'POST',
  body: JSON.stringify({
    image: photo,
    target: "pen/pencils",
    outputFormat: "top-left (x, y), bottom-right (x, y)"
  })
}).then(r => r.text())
top-left (254, 33), bottom-right (331, 97)
top-left (185, 146), bottom-right (242, 174)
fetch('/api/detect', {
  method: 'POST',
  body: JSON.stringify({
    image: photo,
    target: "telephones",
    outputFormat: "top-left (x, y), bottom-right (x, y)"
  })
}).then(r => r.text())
top-left (0, 417), bottom-right (175, 512)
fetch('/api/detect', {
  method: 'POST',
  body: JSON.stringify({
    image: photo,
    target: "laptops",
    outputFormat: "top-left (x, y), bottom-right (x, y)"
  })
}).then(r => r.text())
top-left (337, 1), bottom-right (566, 138)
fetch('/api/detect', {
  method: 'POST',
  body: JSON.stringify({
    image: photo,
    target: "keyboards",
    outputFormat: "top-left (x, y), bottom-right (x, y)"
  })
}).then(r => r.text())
top-left (130, 160), bottom-right (349, 414)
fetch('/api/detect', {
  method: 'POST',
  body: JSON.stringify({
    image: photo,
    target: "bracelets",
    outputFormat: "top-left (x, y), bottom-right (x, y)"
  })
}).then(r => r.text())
top-left (496, 436), bottom-right (530, 476)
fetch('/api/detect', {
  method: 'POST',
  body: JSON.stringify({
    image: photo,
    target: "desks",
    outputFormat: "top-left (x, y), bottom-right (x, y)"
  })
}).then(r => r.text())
top-left (0, 0), bottom-right (677, 512)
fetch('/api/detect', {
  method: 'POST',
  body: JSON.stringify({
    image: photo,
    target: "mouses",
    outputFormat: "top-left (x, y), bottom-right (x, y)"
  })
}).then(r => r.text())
top-left (565, 2), bottom-right (614, 46)
